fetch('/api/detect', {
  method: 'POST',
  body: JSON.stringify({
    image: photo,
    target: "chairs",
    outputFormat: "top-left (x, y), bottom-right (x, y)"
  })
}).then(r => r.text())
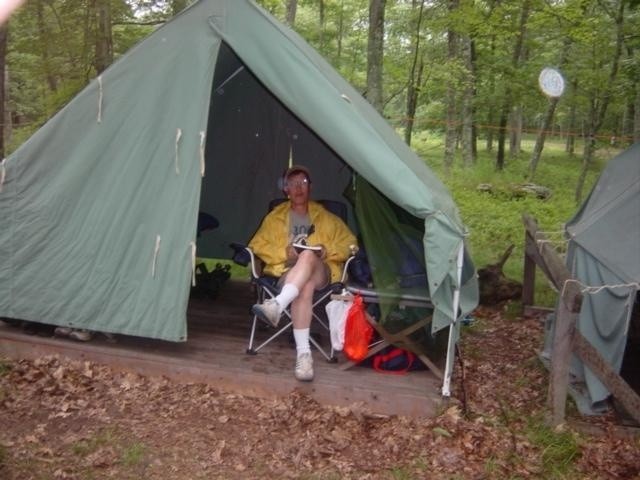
top-left (228, 195), bottom-right (351, 364)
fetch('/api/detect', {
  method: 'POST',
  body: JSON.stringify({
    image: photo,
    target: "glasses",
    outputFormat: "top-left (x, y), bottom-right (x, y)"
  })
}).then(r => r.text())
top-left (286, 178), bottom-right (309, 187)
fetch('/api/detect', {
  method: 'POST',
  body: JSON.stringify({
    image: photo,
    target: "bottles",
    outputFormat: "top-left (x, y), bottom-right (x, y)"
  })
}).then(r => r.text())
top-left (212, 261), bottom-right (231, 278)
top-left (241, 282), bottom-right (257, 315)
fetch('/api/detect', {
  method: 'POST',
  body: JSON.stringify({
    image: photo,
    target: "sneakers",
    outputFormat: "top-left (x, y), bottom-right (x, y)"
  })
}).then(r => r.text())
top-left (250, 297), bottom-right (283, 331)
top-left (292, 345), bottom-right (316, 382)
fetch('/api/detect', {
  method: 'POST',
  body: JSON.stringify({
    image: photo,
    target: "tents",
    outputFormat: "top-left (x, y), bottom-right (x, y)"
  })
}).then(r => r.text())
top-left (536, 139), bottom-right (639, 418)
top-left (0, 0), bottom-right (481, 397)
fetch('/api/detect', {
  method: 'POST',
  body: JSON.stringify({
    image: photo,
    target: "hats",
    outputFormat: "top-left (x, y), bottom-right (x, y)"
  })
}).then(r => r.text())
top-left (282, 164), bottom-right (313, 186)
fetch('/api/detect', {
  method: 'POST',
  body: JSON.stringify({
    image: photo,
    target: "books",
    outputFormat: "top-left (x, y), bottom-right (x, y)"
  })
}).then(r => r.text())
top-left (293, 243), bottom-right (322, 255)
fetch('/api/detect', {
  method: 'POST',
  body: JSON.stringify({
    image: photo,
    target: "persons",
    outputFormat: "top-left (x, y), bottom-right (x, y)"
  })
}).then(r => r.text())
top-left (246, 165), bottom-right (362, 383)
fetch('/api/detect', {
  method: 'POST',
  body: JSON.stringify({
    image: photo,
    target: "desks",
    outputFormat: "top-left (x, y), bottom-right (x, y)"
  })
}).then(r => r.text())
top-left (340, 280), bottom-right (445, 379)
